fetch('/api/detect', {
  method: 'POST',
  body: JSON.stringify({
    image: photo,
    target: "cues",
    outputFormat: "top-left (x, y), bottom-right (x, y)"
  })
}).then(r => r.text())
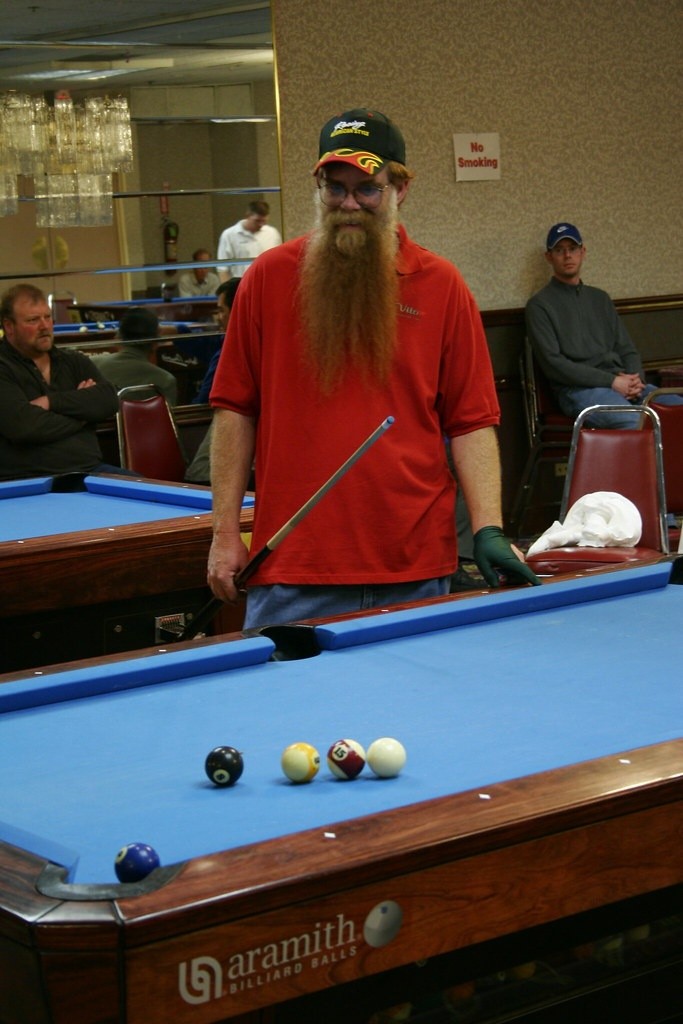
top-left (175, 416), bottom-right (396, 644)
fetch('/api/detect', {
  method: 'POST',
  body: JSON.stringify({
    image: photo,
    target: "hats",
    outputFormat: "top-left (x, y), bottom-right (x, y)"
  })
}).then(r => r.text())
top-left (309, 108), bottom-right (406, 173)
top-left (547, 222), bottom-right (582, 249)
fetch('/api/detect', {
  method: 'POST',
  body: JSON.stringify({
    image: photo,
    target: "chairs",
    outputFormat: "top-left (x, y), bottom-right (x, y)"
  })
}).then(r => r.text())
top-left (636, 387), bottom-right (683, 553)
top-left (517, 351), bottom-right (573, 538)
top-left (501, 404), bottom-right (671, 584)
top-left (511, 335), bottom-right (576, 522)
top-left (115, 383), bottom-right (193, 483)
top-left (47, 290), bottom-right (79, 324)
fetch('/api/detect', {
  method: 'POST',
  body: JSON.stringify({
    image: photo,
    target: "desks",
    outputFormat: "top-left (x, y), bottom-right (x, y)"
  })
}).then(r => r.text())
top-left (67, 295), bottom-right (220, 322)
top-left (0, 553), bottom-right (683, 1024)
top-left (53, 321), bottom-right (119, 343)
top-left (0, 469), bottom-right (255, 620)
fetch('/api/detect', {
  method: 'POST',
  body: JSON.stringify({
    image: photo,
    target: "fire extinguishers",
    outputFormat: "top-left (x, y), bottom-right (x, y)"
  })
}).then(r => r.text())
top-left (163, 218), bottom-right (180, 270)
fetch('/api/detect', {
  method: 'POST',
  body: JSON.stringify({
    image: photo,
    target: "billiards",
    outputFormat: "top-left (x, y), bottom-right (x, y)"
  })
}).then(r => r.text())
top-left (79, 326), bottom-right (88, 332)
top-left (281, 743), bottom-right (321, 784)
top-left (204, 746), bottom-right (244, 787)
top-left (367, 737), bottom-right (407, 778)
top-left (113, 842), bottom-right (161, 884)
top-left (97, 324), bottom-right (117, 329)
top-left (327, 739), bottom-right (366, 779)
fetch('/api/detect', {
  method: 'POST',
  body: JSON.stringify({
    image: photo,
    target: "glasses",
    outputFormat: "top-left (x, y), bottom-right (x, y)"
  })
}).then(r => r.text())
top-left (313, 175), bottom-right (392, 208)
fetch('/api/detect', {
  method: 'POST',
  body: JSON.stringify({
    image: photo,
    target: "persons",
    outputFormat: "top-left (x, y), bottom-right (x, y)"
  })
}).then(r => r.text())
top-left (184, 411), bottom-right (255, 489)
top-left (0, 283), bottom-right (142, 485)
top-left (190, 277), bottom-right (241, 404)
top-left (216, 199), bottom-right (281, 285)
top-left (87, 308), bottom-right (179, 408)
top-left (206, 109), bottom-right (542, 631)
top-left (524, 223), bottom-right (683, 529)
top-left (179, 249), bottom-right (219, 300)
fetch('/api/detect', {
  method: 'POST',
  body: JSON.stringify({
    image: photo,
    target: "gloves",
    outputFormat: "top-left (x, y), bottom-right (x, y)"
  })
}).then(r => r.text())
top-left (473, 525), bottom-right (543, 590)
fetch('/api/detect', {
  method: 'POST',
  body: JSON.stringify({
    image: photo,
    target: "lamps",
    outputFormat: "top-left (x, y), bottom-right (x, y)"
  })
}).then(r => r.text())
top-left (0, 89), bottom-right (133, 230)
